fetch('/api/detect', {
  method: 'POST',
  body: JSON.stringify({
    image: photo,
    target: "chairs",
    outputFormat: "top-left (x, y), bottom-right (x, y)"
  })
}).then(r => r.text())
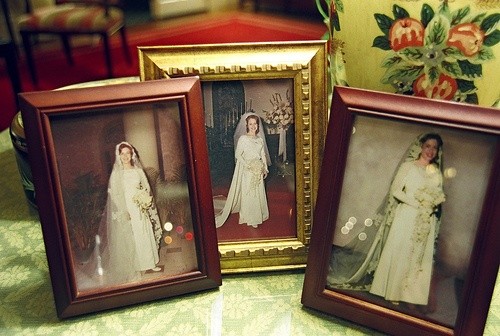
top-left (14, 1), bottom-right (132, 84)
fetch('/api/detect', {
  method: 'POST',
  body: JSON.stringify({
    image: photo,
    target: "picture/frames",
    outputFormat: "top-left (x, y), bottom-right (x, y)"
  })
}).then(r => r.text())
top-left (299, 88), bottom-right (500, 336)
top-left (137, 40), bottom-right (335, 274)
top-left (12, 74), bottom-right (224, 321)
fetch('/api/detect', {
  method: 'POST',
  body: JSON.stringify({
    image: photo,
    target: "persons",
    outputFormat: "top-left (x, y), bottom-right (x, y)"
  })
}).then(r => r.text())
top-left (368, 132), bottom-right (446, 310)
top-left (108, 141), bottom-right (162, 274)
top-left (229, 111), bottom-right (271, 230)
